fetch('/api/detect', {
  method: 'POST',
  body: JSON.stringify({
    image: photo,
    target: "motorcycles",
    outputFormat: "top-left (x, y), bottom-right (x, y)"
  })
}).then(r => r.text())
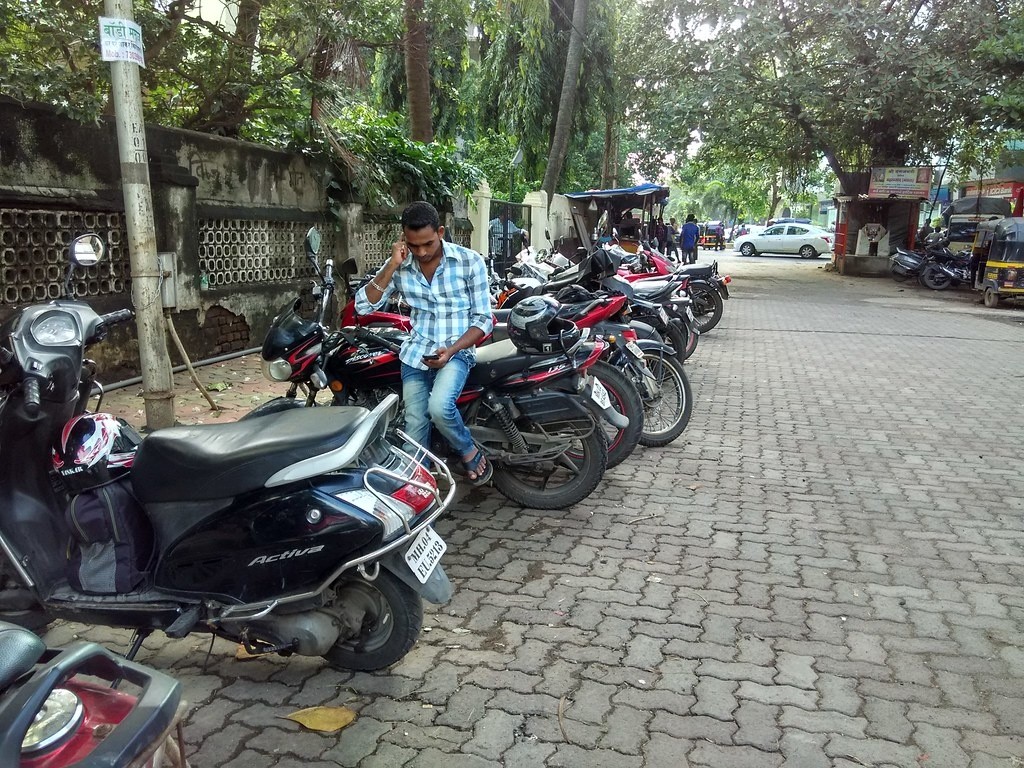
top-left (970, 217), bottom-right (1024, 308)
top-left (890, 238), bottom-right (974, 290)
top-left (337, 212), bottom-right (731, 470)
top-left (239, 227), bottom-right (630, 528)
top-left (0, 233), bottom-right (457, 768)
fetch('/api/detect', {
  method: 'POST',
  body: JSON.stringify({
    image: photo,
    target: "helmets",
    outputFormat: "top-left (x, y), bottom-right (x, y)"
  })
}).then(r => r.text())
top-left (506, 294), bottom-right (581, 353)
top-left (51, 411), bottom-right (138, 492)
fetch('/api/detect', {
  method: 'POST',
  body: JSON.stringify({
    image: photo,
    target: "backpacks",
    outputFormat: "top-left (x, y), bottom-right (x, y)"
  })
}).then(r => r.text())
top-left (61, 487), bottom-right (157, 594)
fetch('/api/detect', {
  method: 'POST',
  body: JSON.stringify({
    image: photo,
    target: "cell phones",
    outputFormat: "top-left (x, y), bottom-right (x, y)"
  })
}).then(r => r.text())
top-left (422, 354), bottom-right (439, 361)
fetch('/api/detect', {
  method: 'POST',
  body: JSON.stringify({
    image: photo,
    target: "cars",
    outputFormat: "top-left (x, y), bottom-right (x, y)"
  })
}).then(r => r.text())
top-left (733, 223), bottom-right (832, 259)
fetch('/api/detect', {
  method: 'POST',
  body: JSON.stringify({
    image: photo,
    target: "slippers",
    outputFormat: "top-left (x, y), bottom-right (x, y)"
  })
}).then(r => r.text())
top-left (461, 440), bottom-right (493, 485)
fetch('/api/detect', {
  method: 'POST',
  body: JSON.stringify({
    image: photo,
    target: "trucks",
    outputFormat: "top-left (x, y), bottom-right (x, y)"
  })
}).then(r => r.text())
top-left (942, 196), bottom-right (1011, 253)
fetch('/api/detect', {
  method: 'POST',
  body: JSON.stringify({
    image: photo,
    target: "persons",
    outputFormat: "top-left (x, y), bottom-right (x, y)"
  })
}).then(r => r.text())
top-left (704, 221), bottom-right (749, 251)
top-left (918, 218), bottom-right (945, 243)
top-left (830, 221), bottom-right (835, 232)
top-left (620, 212), bottom-right (700, 265)
top-left (354, 202), bottom-right (493, 487)
top-left (489, 206), bottom-right (529, 261)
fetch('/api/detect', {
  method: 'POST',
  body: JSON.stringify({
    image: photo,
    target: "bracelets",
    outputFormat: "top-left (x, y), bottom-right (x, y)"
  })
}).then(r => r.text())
top-left (370, 279), bottom-right (386, 293)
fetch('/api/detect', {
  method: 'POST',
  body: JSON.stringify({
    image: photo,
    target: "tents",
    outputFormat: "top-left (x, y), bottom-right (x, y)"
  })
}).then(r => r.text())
top-left (564, 183), bottom-right (670, 242)
